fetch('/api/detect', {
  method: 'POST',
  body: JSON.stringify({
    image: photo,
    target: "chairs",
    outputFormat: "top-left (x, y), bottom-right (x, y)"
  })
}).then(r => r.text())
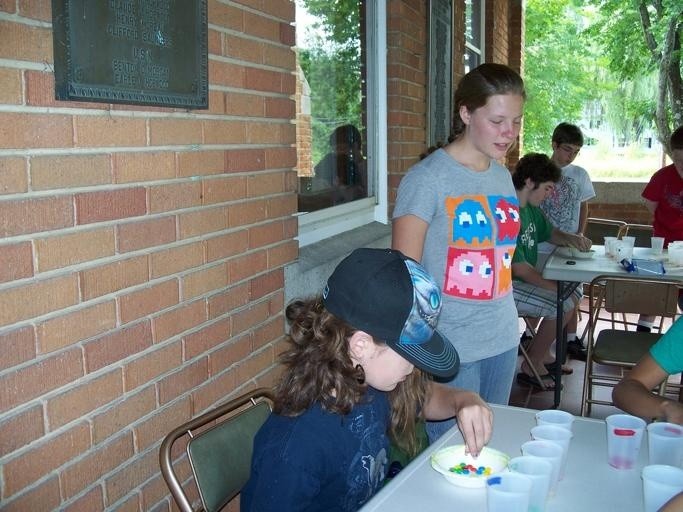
top-left (514, 217), bottom-right (682, 423)
top-left (157, 386), bottom-right (275, 512)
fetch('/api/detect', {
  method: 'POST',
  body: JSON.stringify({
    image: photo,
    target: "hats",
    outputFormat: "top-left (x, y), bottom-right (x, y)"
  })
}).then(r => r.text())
top-left (322, 248), bottom-right (460, 378)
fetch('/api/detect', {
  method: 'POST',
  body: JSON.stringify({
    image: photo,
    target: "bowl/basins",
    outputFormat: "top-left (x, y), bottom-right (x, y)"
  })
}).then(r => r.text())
top-left (570, 248), bottom-right (596, 259)
top-left (431, 445), bottom-right (510, 488)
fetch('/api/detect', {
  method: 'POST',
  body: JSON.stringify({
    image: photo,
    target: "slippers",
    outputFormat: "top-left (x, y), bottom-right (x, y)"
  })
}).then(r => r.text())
top-left (517, 373), bottom-right (564, 392)
top-left (544, 360), bottom-right (573, 375)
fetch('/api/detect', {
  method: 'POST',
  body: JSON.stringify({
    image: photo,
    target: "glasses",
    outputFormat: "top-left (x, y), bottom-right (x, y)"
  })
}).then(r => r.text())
top-left (558, 146), bottom-right (580, 156)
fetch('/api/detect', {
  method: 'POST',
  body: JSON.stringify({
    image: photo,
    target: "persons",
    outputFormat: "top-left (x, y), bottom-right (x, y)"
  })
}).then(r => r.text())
top-left (519, 122), bottom-right (598, 356)
top-left (237, 248), bottom-right (495, 510)
top-left (608, 311), bottom-right (681, 426)
top-left (308, 152), bottom-right (368, 205)
top-left (329, 125), bottom-right (368, 188)
top-left (510, 152), bottom-right (593, 393)
top-left (393, 63), bottom-right (524, 447)
top-left (634, 128), bottom-right (682, 333)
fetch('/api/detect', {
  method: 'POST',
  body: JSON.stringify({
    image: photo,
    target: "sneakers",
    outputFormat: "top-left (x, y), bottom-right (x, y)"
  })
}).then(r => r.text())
top-left (566, 336), bottom-right (588, 361)
top-left (518, 331), bottom-right (533, 355)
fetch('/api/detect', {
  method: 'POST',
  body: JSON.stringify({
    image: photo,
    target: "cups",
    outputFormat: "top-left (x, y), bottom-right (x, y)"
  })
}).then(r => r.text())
top-left (604, 414), bottom-right (646, 470)
top-left (640, 464), bottom-right (683, 512)
top-left (603, 236), bottom-right (683, 266)
top-left (646, 421), bottom-right (683, 468)
top-left (485, 408), bottom-right (577, 512)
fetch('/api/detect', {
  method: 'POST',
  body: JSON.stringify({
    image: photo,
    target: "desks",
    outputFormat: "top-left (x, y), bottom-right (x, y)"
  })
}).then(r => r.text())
top-left (356, 400), bottom-right (651, 511)
